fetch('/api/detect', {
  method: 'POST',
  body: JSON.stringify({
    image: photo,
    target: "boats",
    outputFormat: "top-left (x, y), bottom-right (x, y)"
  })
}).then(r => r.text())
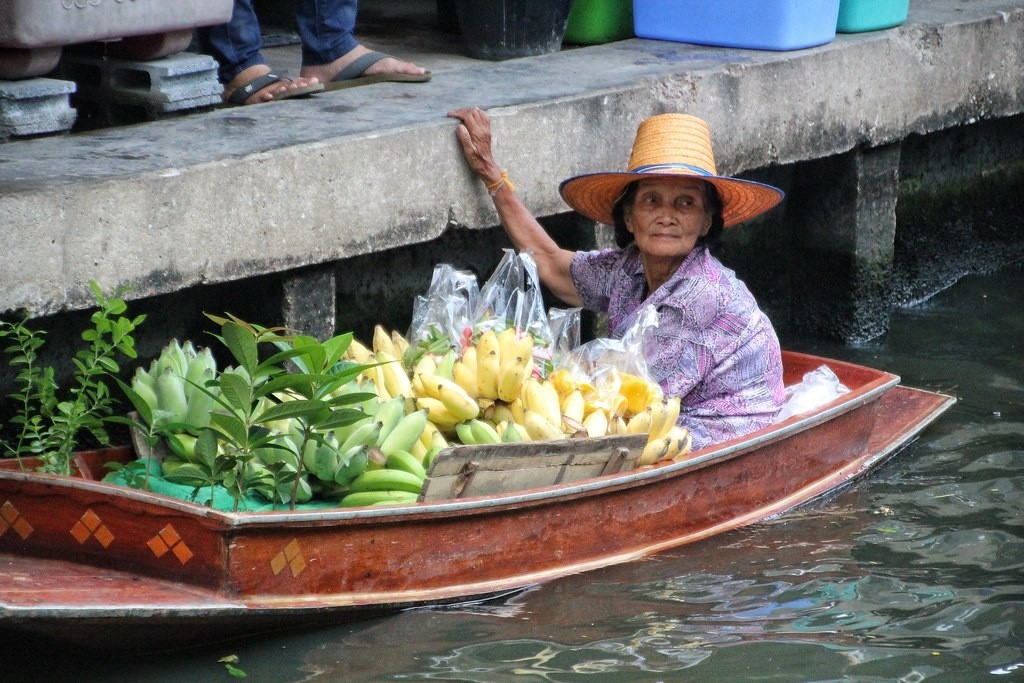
top-left (0, 348), bottom-right (958, 625)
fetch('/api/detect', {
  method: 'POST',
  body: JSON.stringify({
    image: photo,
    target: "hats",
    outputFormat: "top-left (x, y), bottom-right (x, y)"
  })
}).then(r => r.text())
top-left (560, 113), bottom-right (785, 228)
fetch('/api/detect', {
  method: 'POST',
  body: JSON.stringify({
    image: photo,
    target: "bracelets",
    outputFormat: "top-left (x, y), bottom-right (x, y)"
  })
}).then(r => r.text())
top-left (487, 171), bottom-right (515, 197)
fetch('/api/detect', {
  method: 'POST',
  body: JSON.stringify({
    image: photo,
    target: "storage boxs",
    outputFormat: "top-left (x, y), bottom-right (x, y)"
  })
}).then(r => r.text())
top-left (438, 0), bottom-right (570, 62)
top-left (632, 0), bottom-right (841, 51)
top-left (837, 0), bottom-right (910, 34)
top-left (563, 0), bottom-right (636, 45)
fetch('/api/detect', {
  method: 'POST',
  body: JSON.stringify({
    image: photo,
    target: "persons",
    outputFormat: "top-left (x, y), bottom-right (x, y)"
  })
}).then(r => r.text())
top-left (197, 0), bottom-right (433, 106)
top-left (447, 106), bottom-right (785, 452)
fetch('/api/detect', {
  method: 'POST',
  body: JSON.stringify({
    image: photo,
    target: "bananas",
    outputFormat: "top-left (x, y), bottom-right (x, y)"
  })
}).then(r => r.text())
top-left (131, 323), bottom-right (690, 507)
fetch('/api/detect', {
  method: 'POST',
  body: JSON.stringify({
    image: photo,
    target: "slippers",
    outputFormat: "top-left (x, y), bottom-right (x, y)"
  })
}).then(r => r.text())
top-left (325, 51), bottom-right (432, 91)
top-left (230, 65), bottom-right (326, 105)
